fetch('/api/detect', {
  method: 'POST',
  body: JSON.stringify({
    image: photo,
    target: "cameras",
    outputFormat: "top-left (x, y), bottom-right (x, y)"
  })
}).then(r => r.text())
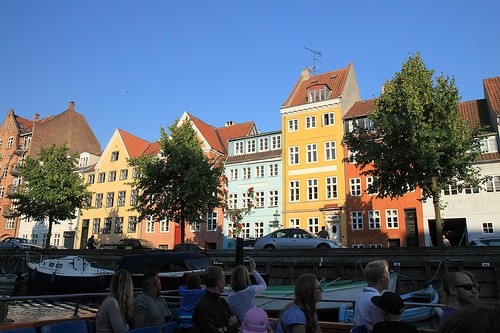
top-left (243, 260), bottom-right (250, 265)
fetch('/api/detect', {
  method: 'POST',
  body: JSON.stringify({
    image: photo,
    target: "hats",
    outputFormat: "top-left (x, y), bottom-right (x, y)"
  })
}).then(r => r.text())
top-left (371, 292), bottom-right (405, 314)
top-left (242, 308), bottom-right (269, 333)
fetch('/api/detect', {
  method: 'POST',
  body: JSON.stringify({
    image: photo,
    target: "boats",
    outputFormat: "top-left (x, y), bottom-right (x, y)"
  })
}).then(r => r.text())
top-left (22, 249), bottom-right (116, 293)
top-left (100, 251), bottom-right (440, 325)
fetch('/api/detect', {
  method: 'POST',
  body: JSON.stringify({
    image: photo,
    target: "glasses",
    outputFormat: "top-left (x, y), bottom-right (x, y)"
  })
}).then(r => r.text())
top-left (144, 271), bottom-right (158, 279)
top-left (127, 269), bottom-right (132, 275)
top-left (314, 286), bottom-right (323, 292)
top-left (452, 283), bottom-right (481, 292)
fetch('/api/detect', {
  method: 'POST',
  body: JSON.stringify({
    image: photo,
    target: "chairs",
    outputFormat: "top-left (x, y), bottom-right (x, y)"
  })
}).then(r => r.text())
top-left (350, 324), bottom-right (368, 333)
top-left (0, 319), bottom-right (192, 333)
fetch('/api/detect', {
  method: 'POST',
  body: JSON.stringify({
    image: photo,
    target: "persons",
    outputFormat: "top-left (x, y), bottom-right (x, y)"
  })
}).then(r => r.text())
top-left (192, 266), bottom-right (242, 333)
top-left (442, 235), bottom-right (451, 247)
top-left (275, 273), bottom-right (322, 333)
top-left (317, 226), bottom-right (329, 240)
top-left (87, 235), bottom-right (98, 249)
top-left (436, 270), bottom-right (500, 333)
top-left (370, 292), bottom-right (420, 333)
top-left (237, 307), bottom-right (274, 333)
top-left (353, 259), bottom-right (390, 333)
top-left (228, 259), bottom-right (267, 323)
top-left (95, 269), bottom-right (134, 333)
top-left (178, 272), bottom-right (207, 333)
top-left (133, 272), bottom-right (174, 333)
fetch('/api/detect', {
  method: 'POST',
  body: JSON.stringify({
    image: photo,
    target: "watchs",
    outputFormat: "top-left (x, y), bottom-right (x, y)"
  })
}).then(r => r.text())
top-left (222, 326), bottom-right (227, 333)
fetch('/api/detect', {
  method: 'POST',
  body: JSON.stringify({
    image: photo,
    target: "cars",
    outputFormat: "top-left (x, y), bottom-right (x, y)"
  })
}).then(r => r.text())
top-left (467, 236), bottom-right (500, 246)
top-left (173, 243), bottom-right (206, 252)
top-left (116, 238), bottom-right (163, 254)
top-left (254, 226), bottom-right (342, 249)
top-left (0, 236), bottom-right (44, 249)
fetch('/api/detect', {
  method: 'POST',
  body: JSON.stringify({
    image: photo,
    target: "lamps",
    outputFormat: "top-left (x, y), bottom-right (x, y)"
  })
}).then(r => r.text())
top-left (273, 210), bottom-right (281, 225)
